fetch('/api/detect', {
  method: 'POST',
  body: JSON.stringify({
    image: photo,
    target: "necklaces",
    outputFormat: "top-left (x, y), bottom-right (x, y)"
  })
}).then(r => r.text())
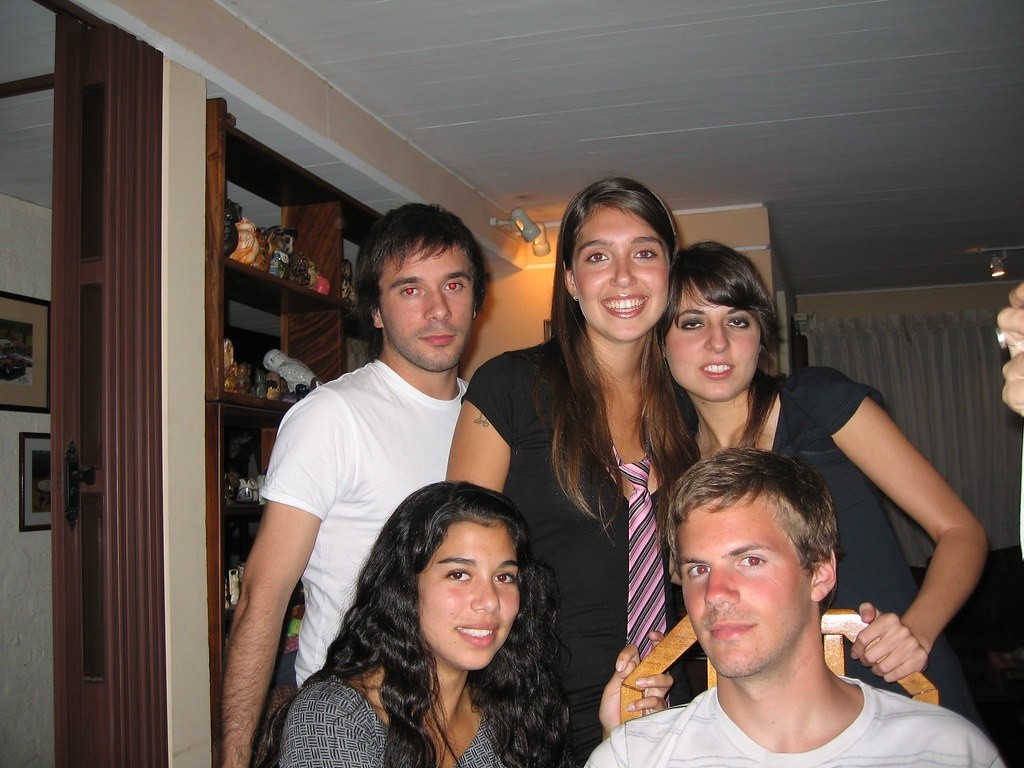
top-left (698, 421), bottom-right (712, 455)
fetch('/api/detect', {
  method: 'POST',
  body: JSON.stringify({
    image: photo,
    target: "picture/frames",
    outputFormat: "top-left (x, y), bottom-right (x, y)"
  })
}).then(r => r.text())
top-left (0, 291), bottom-right (52, 414)
top-left (18, 432), bottom-right (51, 532)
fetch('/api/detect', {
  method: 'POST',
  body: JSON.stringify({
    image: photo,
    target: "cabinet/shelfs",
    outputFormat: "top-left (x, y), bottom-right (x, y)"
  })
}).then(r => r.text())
top-left (199, 94), bottom-right (396, 758)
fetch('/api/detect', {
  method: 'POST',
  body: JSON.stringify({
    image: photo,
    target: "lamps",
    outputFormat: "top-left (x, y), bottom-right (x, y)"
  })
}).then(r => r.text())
top-left (530, 221), bottom-right (550, 258)
top-left (488, 208), bottom-right (541, 243)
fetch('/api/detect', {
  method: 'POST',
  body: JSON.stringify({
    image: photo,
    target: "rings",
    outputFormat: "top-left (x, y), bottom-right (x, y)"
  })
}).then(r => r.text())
top-left (995, 328), bottom-right (1024, 349)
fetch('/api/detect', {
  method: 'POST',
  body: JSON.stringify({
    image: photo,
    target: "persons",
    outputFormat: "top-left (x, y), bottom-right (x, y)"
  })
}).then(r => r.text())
top-left (222, 202), bottom-right (487, 768)
top-left (445, 174), bottom-right (1024, 768)
top-left (255, 481), bottom-right (570, 768)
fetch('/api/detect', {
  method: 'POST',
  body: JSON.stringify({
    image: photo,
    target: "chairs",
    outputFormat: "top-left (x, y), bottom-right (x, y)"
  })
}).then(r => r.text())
top-left (618, 608), bottom-right (938, 722)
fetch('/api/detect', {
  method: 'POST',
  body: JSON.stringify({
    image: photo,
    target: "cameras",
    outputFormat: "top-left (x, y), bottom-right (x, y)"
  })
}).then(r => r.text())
top-left (995, 329), bottom-right (1008, 350)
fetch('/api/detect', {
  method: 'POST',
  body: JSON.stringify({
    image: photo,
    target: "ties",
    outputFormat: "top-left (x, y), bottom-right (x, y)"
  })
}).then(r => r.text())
top-left (610, 436), bottom-right (670, 710)
